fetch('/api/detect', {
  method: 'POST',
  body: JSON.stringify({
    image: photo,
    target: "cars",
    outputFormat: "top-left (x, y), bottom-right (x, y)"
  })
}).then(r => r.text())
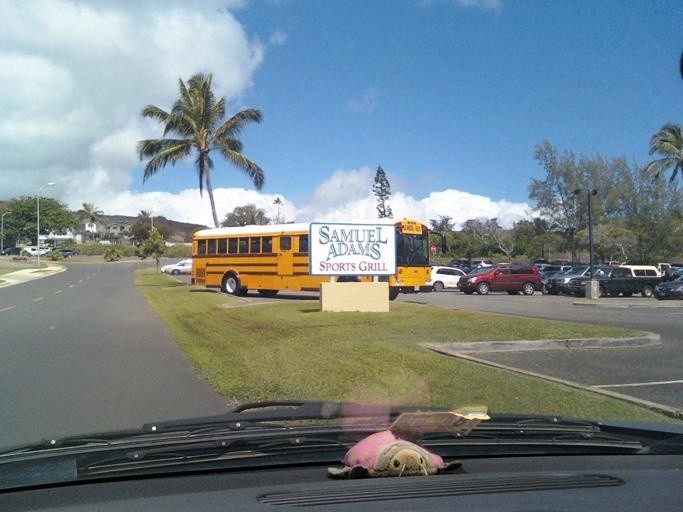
top-left (1, 246), bottom-right (75, 260)
top-left (418, 257), bottom-right (683, 301)
top-left (159, 256), bottom-right (191, 275)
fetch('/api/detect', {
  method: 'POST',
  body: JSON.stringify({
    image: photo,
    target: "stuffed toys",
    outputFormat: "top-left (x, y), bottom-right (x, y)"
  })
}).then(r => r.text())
top-left (326, 427), bottom-right (464, 479)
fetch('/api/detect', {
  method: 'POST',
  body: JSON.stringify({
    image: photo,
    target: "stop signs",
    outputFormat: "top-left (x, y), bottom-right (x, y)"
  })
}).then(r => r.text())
top-left (429, 245), bottom-right (436, 252)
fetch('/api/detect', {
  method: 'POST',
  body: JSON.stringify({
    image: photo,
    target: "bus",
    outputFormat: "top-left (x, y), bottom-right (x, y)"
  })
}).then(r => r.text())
top-left (192, 220), bottom-right (447, 302)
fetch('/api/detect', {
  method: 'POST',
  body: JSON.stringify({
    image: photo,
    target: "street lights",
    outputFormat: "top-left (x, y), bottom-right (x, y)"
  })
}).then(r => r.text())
top-left (35, 182), bottom-right (55, 266)
top-left (573, 187), bottom-right (599, 300)
top-left (0, 211), bottom-right (12, 254)
